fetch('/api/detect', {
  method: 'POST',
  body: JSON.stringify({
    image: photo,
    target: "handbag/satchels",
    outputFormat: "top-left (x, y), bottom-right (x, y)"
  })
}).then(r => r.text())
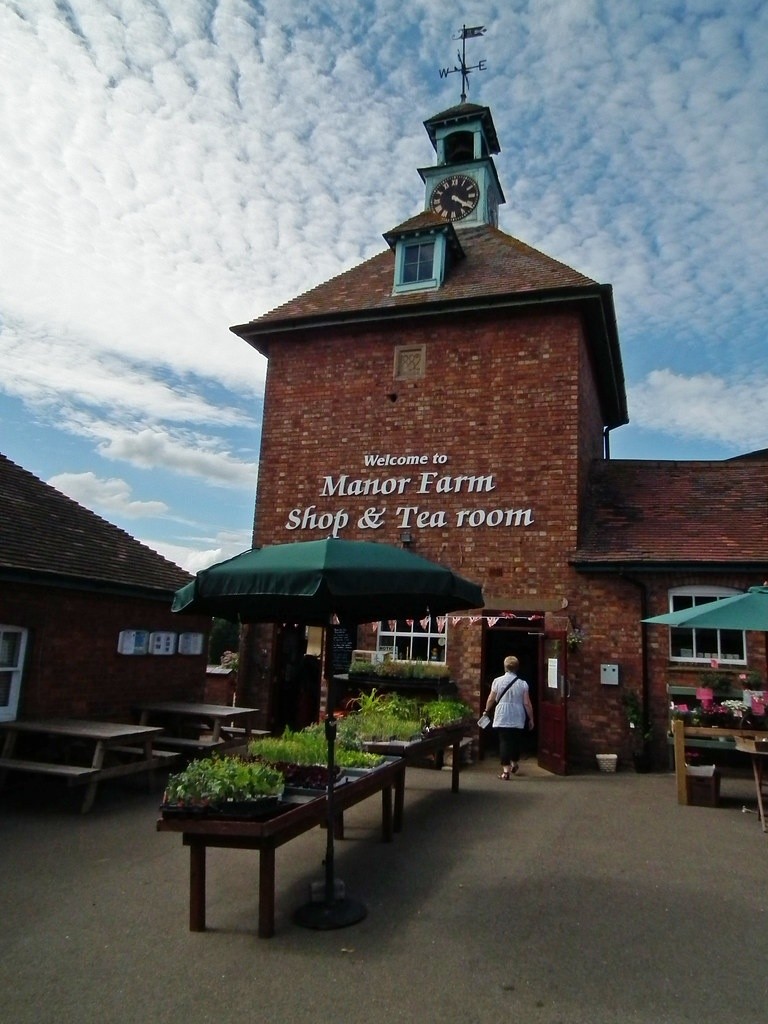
top-left (487, 700), bottom-right (498, 725)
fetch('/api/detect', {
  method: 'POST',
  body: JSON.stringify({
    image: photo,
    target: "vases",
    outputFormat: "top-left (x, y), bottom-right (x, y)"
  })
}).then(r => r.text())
top-left (685, 719), bottom-right (768, 731)
top-left (596, 753), bottom-right (618, 772)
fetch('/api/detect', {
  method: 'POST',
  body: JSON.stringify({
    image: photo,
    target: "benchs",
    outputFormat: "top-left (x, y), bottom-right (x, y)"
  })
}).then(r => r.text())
top-left (152, 734), bottom-right (224, 762)
top-left (187, 721), bottom-right (273, 744)
top-left (106, 743), bottom-right (181, 795)
top-left (2, 758), bottom-right (102, 817)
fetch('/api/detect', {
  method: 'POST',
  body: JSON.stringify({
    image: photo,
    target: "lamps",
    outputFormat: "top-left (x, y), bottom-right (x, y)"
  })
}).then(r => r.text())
top-left (400, 532), bottom-right (414, 543)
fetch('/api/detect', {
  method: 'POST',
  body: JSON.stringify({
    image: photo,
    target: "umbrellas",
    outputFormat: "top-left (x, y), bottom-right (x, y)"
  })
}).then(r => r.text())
top-left (639, 581), bottom-right (768, 631)
top-left (170, 536), bottom-right (486, 931)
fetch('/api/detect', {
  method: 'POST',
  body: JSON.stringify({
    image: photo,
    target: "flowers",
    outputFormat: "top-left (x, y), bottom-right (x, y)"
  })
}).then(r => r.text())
top-left (567, 626), bottom-right (589, 647)
top-left (668, 700), bottom-right (768, 722)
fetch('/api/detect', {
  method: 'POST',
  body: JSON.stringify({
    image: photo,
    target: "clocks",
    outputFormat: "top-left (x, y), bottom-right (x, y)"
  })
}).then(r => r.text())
top-left (430, 175), bottom-right (480, 223)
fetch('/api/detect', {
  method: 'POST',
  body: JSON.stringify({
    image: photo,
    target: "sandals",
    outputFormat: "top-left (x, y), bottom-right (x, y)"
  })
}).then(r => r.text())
top-left (511, 764), bottom-right (519, 773)
top-left (497, 772), bottom-right (510, 780)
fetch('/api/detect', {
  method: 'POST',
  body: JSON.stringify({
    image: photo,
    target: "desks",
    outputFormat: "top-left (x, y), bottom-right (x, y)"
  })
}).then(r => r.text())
top-left (360, 729), bottom-right (465, 823)
top-left (155, 752), bottom-right (406, 939)
top-left (0, 714), bottom-right (164, 815)
top-left (129, 699), bottom-right (261, 762)
top-left (668, 719), bottom-right (768, 806)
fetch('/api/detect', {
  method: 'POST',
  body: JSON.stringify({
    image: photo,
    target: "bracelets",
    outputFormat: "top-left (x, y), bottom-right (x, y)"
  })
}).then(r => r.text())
top-left (484, 709), bottom-right (488, 712)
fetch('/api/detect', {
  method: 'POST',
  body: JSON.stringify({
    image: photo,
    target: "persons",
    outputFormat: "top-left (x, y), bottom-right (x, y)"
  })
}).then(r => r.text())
top-left (483, 655), bottom-right (534, 779)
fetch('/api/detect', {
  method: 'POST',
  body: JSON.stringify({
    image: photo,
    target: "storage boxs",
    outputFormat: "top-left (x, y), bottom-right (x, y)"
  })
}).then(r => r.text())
top-left (684, 770), bottom-right (720, 806)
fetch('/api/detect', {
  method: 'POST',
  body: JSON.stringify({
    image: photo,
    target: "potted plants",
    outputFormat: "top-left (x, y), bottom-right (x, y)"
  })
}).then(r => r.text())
top-left (158, 756), bottom-right (285, 821)
top-left (347, 659), bottom-right (453, 685)
top-left (621, 690), bottom-right (659, 774)
top-left (736, 671), bottom-right (760, 691)
